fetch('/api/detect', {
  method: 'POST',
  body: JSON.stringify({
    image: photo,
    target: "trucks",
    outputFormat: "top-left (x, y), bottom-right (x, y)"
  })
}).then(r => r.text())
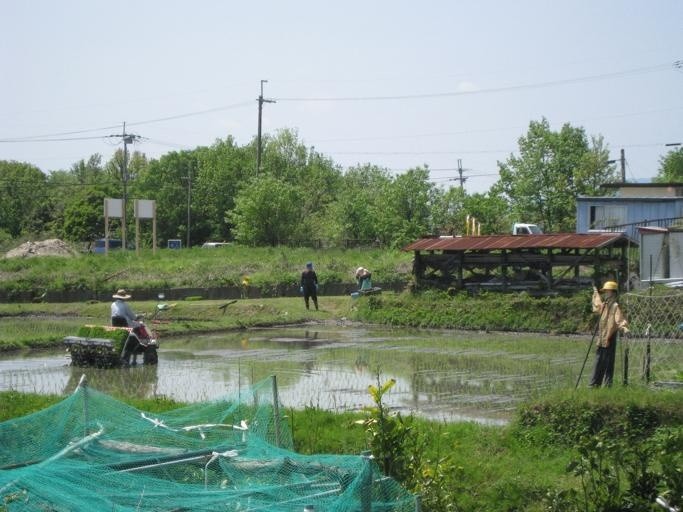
top-left (512, 223), bottom-right (544, 235)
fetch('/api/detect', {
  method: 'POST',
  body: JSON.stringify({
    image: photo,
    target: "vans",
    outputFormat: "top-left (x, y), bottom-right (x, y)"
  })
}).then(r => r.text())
top-left (94, 238), bottom-right (136, 254)
top-left (200, 242), bottom-right (230, 250)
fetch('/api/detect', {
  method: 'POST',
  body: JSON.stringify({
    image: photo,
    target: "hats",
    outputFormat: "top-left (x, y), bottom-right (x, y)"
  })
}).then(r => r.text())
top-left (599, 282), bottom-right (617, 292)
top-left (112, 290), bottom-right (130, 299)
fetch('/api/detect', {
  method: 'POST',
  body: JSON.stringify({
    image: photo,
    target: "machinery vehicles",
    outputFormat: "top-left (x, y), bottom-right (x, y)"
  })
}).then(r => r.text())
top-left (63, 315), bottom-right (159, 369)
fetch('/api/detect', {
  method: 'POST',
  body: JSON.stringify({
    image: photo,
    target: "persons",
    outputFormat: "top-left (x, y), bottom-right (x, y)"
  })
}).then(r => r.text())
top-left (300, 262), bottom-right (319, 311)
top-left (585, 277), bottom-right (632, 388)
top-left (240, 276), bottom-right (250, 299)
top-left (590, 262), bottom-right (639, 295)
top-left (111, 288), bottom-right (141, 334)
top-left (354, 267), bottom-right (372, 290)
